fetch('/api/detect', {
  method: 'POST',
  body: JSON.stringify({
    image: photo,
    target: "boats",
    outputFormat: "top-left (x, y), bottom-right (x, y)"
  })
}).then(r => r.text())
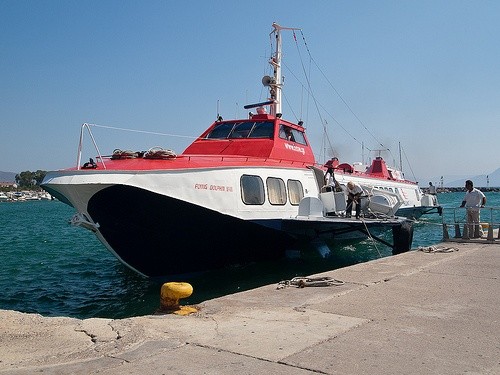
top-left (0, 188), bottom-right (55, 201)
top-left (39, 21), bottom-right (443, 290)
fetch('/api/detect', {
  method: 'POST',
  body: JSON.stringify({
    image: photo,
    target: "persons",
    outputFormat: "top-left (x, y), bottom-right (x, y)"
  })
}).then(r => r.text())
top-left (459, 180), bottom-right (487, 238)
top-left (343, 180), bottom-right (364, 219)
top-left (425, 182), bottom-right (437, 206)
